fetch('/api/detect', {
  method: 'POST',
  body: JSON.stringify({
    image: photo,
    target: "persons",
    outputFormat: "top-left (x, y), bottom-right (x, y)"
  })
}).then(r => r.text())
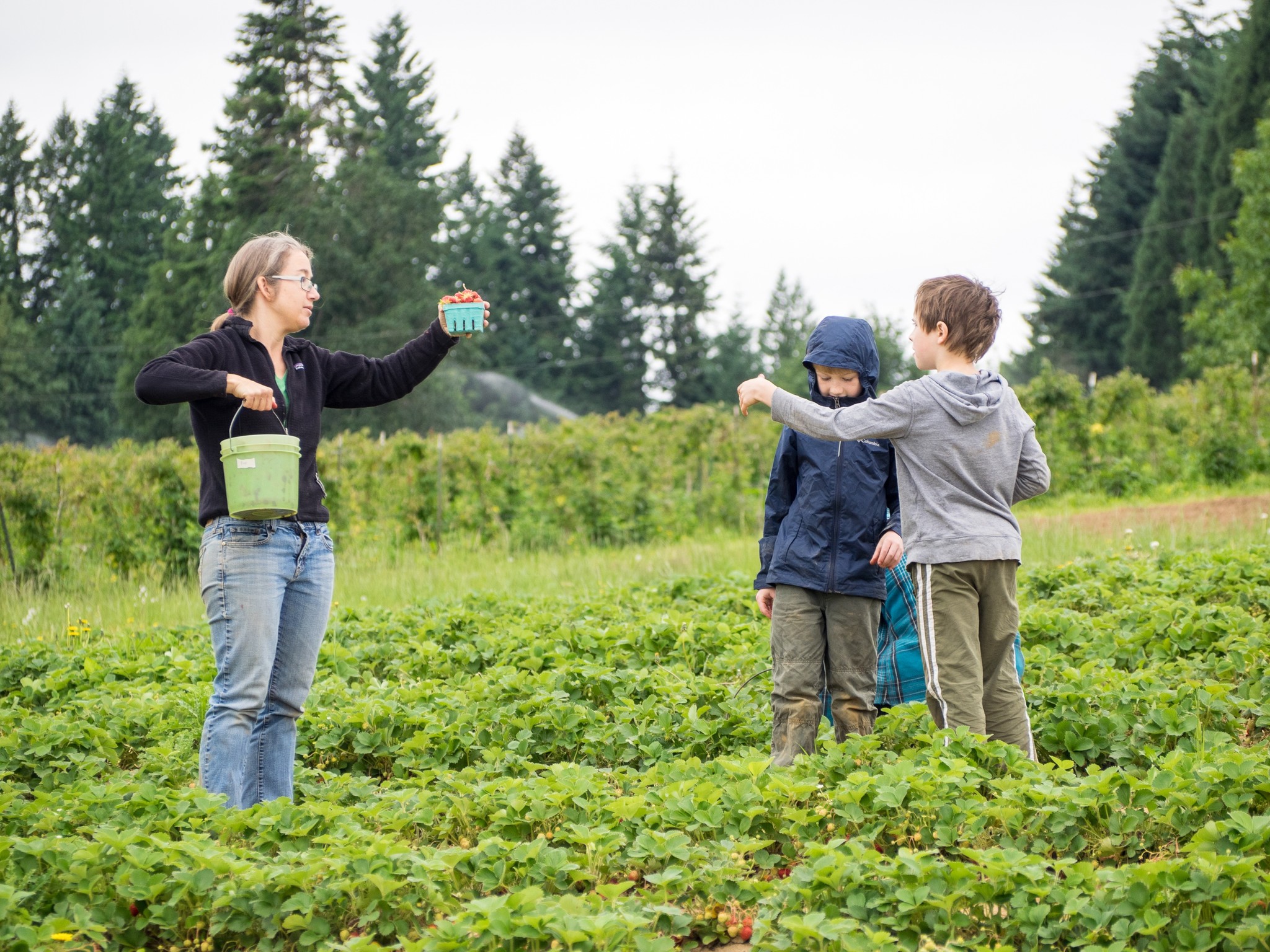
top-left (755, 316), bottom-right (903, 764)
top-left (738, 276), bottom-right (1052, 762)
top-left (134, 224), bottom-right (490, 808)
top-left (817, 506), bottom-right (1025, 728)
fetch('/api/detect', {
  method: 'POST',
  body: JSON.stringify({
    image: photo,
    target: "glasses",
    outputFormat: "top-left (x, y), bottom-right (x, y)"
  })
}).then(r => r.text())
top-left (268, 275), bottom-right (318, 293)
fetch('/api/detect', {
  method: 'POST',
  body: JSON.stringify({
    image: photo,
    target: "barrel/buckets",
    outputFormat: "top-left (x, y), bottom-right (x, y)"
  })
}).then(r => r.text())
top-left (220, 398), bottom-right (302, 519)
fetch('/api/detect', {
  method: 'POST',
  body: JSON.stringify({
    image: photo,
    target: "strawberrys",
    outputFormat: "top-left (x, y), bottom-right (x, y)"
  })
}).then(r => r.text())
top-left (331, 823), bottom-right (885, 943)
top-left (441, 284), bottom-right (483, 304)
